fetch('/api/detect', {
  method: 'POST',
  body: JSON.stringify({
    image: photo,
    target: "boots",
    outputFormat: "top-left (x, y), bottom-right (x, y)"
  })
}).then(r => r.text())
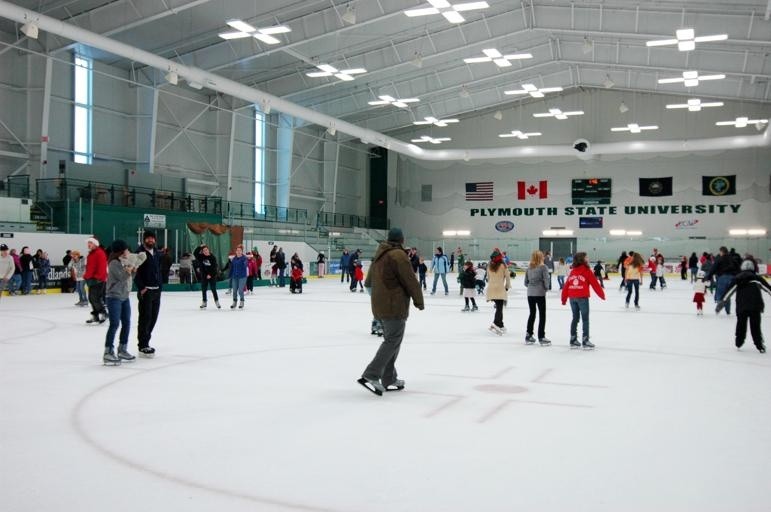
top-left (525, 332), bottom-right (535, 342)
top-left (230, 301), bottom-right (236, 308)
top-left (118, 342), bottom-right (135, 360)
top-left (376, 321), bottom-right (383, 333)
top-left (214, 301), bottom-right (220, 308)
top-left (238, 301), bottom-right (243, 307)
top-left (491, 323), bottom-right (503, 334)
top-left (538, 332), bottom-right (550, 343)
top-left (371, 320), bottom-right (376, 331)
top-left (471, 304), bottom-right (478, 311)
top-left (582, 335), bottom-right (595, 347)
top-left (570, 335), bottom-right (581, 346)
top-left (199, 301), bottom-right (206, 308)
top-left (103, 345), bottom-right (121, 361)
top-left (461, 305), bottom-right (470, 311)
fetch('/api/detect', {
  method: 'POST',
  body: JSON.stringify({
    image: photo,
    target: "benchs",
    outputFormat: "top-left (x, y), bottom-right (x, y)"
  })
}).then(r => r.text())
top-left (223, 219), bottom-right (381, 262)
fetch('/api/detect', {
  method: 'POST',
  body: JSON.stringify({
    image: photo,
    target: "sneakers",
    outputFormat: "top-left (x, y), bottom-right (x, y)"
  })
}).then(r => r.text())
top-left (390, 380), bottom-right (404, 387)
top-left (75, 300), bottom-right (107, 324)
top-left (362, 374), bottom-right (386, 392)
top-left (8, 288), bottom-right (45, 296)
top-left (138, 345), bottom-right (155, 353)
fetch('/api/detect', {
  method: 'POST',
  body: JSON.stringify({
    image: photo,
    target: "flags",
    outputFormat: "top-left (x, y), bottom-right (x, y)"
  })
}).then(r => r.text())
top-left (517, 181), bottom-right (548, 199)
top-left (702, 175), bottom-right (737, 196)
top-left (464, 182), bottom-right (494, 201)
top-left (639, 178), bottom-right (672, 197)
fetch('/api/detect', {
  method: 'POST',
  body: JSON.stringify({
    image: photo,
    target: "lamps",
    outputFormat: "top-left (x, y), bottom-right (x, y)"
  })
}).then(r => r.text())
top-left (658, 70), bottom-right (727, 88)
top-left (463, 47), bottom-right (533, 67)
top-left (493, 109), bottom-right (503, 121)
top-left (582, 39), bottom-right (593, 54)
top-left (610, 124), bottom-right (659, 134)
top-left (413, 53), bottom-right (423, 69)
top-left (327, 123), bottom-right (337, 137)
top-left (219, 18), bottom-right (292, 45)
top-left (665, 99), bottom-right (725, 113)
top-left (619, 102), bottom-right (628, 114)
top-left (532, 109), bottom-right (584, 120)
top-left (305, 63), bottom-right (367, 81)
top-left (20, 21), bottom-right (39, 40)
top-left (410, 135), bottom-right (453, 145)
top-left (498, 128), bottom-right (542, 140)
top-left (402, 1), bottom-right (490, 23)
top-left (413, 116), bottom-right (460, 128)
top-left (603, 77), bottom-right (615, 89)
top-left (367, 94), bottom-right (421, 109)
top-left (714, 114), bottom-right (768, 129)
top-left (505, 83), bottom-right (563, 98)
top-left (165, 71), bottom-right (178, 85)
top-left (360, 129), bottom-right (372, 145)
top-left (260, 99), bottom-right (271, 115)
top-left (341, 4), bottom-right (357, 24)
top-left (459, 86), bottom-right (468, 99)
top-left (646, 29), bottom-right (729, 51)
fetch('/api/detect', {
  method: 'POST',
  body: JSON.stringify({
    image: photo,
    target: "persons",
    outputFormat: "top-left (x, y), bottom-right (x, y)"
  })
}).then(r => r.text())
top-left (364, 226), bottom-right (426, 391)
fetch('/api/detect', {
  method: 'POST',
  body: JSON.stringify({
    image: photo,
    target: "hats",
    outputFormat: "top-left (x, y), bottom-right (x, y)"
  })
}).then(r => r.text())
top-left (143, 230), bottom-right (156, 240)
top-left (740, 260), bottom-right (754, 271)
top-left (87, 236), bottom-right (98, 246)
top-left (0, 244), bottom-right (8, 250)
top-left (696, 270), bottom-right (704, 279)
top-left (387, 227), bottom-right (403, 242)
top-left (490, 251), bottom-right (501, 262)
top-left (111, 240), bottom-right (128, 253)
